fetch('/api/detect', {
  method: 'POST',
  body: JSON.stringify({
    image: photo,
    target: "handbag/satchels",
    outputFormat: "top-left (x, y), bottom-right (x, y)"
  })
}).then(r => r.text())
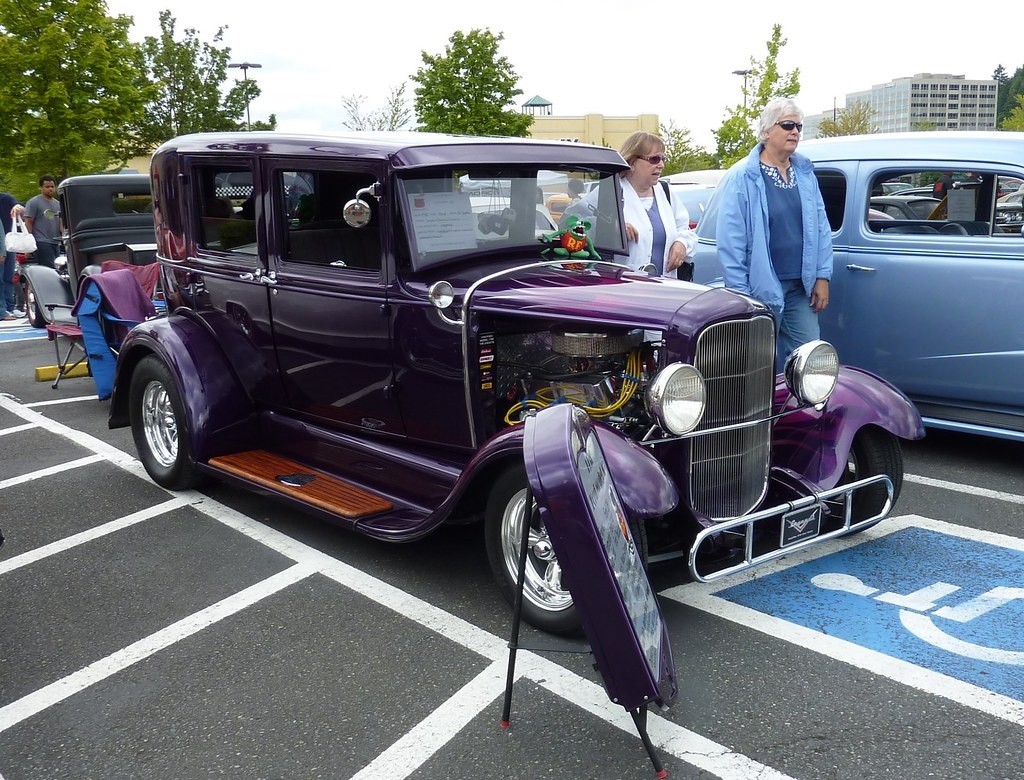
top-left (5, 214), bottom-right (37, 252)
top-left (659, 181), bottom-right (694, 281)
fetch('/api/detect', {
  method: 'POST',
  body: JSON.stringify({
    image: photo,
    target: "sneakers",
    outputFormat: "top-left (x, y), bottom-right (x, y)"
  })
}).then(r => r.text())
top-left (6, 310), bottom-right (26, 318)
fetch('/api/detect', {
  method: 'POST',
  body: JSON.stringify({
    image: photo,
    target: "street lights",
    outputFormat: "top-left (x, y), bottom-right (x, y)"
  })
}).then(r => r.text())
top-left (228, 64), bottom-right (262, 131)
top-left (732, 70), bottom-right (760, 139)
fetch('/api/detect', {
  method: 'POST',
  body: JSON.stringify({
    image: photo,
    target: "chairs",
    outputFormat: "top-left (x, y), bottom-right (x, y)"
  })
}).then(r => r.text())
top-left (940, 221), bottom-right (1004, 236)
top-left (43, 259), bottom-right (161, 389)
top-left (71, 270), bottom-right (157, 402)
top-left (883, 226), bottom-right (940, 235)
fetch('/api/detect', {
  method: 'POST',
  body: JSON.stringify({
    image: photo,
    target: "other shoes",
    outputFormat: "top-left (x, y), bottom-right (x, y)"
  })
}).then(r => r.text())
top-left (0, 316), bottom-right (17, 321)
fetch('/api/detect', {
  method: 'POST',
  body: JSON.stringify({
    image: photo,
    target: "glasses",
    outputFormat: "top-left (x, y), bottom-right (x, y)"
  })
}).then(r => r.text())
top-left (775, 120), bottom-right (802, 132)
top-left (637, 155), bottom-right (665, 164)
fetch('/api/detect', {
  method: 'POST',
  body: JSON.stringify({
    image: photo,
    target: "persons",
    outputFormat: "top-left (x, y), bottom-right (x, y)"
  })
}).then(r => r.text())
top-left (559, 131), bottom-right (699, 279)
top-left (0, 175), bottom-right (64, 321)
top-left (535, 186), bottom-right (543, 205)
top-left (933, 171), bottom-right (1007, 200)
top-left (717, 97), bottom-right (834, 375)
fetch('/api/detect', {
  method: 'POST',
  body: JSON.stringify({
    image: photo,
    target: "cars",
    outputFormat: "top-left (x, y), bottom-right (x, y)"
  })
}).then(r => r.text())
top-left (19, 132), bottom-right (1024, 637)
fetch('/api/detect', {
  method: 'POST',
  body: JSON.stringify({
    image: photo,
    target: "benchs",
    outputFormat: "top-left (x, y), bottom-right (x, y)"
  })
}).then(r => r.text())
top-left (289, 226), bottom-right (381, 267)
